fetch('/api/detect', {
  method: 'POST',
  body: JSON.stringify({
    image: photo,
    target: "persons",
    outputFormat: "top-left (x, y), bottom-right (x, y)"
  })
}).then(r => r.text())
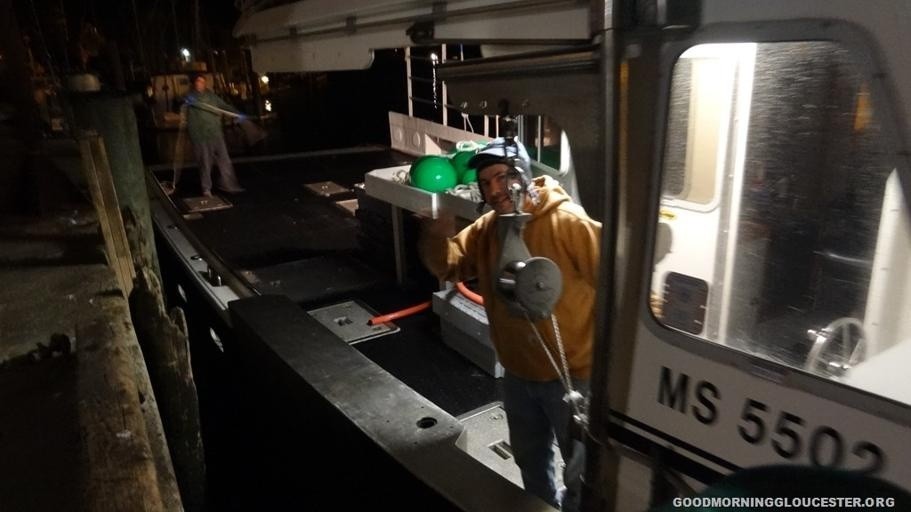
top-left (180, 76), bottom-right (247, 199)
top-left (416, 135), bottom-right (602, 512)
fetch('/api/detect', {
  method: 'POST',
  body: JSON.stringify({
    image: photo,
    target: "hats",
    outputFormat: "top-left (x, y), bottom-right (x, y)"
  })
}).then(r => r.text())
top-left (466, 136), bottom-right (529, 170)
